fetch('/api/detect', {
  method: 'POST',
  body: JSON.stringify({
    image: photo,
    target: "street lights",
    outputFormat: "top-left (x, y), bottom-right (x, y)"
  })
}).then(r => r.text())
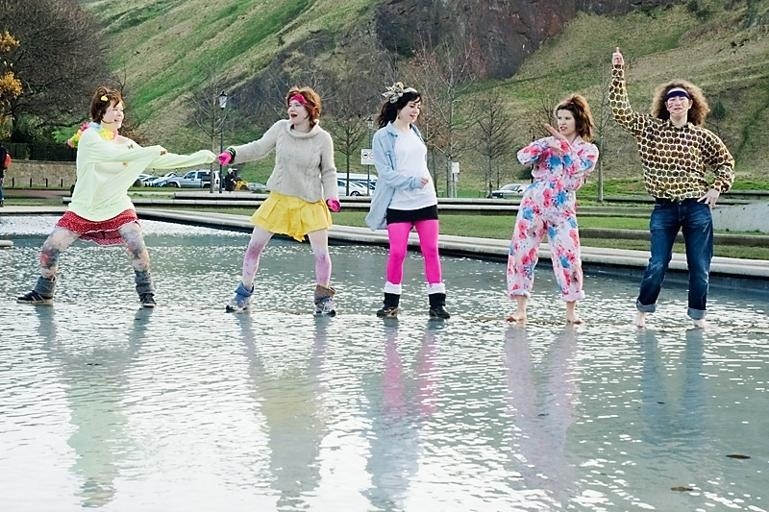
top-left (215, 91), bottom-right (228, 193)
top-left (366, 115), bottom-right (374, 197)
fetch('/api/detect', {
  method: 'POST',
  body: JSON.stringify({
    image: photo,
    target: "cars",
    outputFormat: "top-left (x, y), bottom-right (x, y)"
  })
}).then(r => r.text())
top-left (336, 172), bottom-right (378, 199)
top-left (136, 167), bottom-right (266, 193)
top-left (487, 182), bottom-right (527, 200)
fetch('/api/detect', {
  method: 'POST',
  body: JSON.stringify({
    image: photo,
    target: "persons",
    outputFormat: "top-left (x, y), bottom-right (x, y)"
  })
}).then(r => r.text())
top-left (0, 140), bottom-right (8, 208)
top-left (215, 85), bottom-right (341, 318)
top-left (16, 87), bottom-right (219, 309)
top-left (606, 45), bottom-right (736, 331)
top-left (503, 91), bottom-right (600, 325)
top-left (225, 168), bottom-right (237, 191)
top-left (365, 81), bottom-right (452, 319)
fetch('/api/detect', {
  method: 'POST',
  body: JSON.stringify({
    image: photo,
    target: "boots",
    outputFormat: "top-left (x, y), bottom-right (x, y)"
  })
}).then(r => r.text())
top-left (135, 269), bottom-right (156, 308)
top-left (376, 282), bottom-right (402, 318)
top-left (225, 281), bottom-right (255, 310)
top-left (17, 274), bottom-right (58, 306)
top-left (313, 285), bottom-right (336, 317)
top-left (427, 282), bottom-right (450, 318)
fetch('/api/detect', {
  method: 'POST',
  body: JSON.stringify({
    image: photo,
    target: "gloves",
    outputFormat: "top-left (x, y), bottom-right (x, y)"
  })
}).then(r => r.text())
top-left (217, 151), bottom-right (233, 166)
top-left (327, 198), bottom-right (341, 212)
